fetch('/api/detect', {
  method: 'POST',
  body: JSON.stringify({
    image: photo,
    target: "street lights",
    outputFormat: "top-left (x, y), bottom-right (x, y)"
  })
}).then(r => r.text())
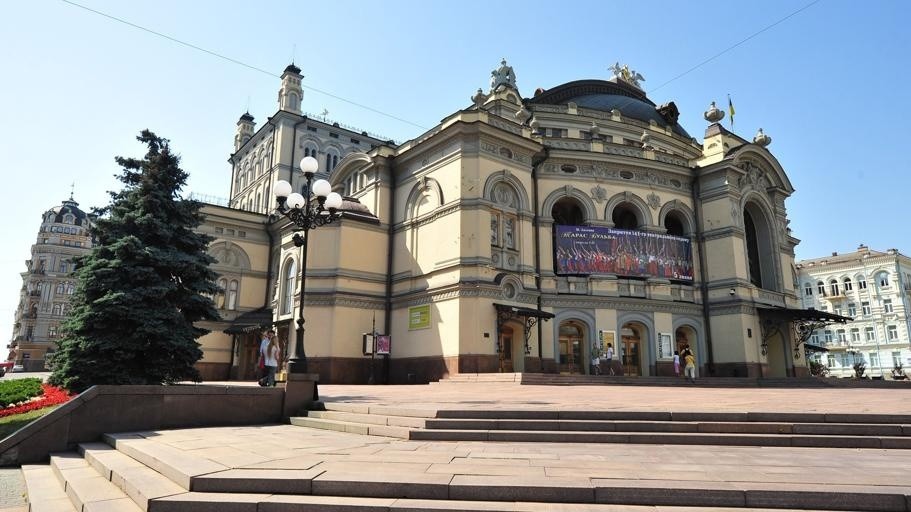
top-left (273, 155), bottom-right (343, 374)
top-left (846, 343), bottom-right (860, 364)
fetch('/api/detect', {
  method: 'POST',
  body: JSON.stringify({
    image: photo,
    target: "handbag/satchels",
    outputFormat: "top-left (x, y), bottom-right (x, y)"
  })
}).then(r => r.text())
top-left (259, 351), bottom-right (265, 369)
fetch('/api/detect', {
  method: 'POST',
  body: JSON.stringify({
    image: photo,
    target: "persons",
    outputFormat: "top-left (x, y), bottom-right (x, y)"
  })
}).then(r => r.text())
top-left (553, 230), bottom-right (693, 279)
top-left (259, 330), bottom-right (276, 387)
top-left (681, 344), bottom-right (695, 377)
top-left (672, 350), bottom-right (681, 377)
top-left (590, 343), bottom-right (604, 376)
top-left (257, 336), bottom-right (280, 387)
top-left (684, 350), bottom-right (696, 383)
top-left (606, 342), bottom-right (617, 377)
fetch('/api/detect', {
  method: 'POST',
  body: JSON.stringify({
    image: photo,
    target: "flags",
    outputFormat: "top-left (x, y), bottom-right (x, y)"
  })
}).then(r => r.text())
top-left (728, 96), bottom-right (736, 127)
